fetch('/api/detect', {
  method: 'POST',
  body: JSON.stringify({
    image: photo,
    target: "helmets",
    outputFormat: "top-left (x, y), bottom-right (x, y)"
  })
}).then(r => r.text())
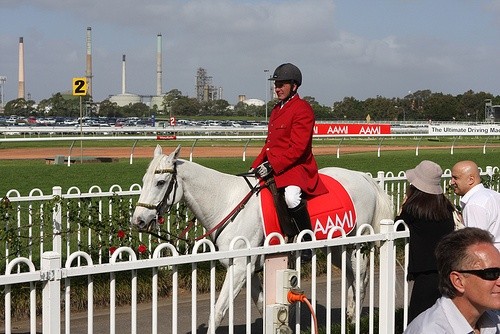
top-left (268, 63), bottom-right (302, 85)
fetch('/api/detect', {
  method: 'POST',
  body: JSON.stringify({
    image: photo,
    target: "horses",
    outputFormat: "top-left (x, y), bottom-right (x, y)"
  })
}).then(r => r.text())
top-left (132, 144), bottom-right (395, 334)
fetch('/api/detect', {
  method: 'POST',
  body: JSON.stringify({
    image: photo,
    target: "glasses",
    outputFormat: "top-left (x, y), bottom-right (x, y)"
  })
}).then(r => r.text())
top-left (457, 267), bottom-right (500, 281)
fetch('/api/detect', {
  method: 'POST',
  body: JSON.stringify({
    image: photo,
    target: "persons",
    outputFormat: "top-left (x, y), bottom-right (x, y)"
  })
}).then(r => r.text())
top-left (395, 159), bottom-right (500, 334)
top-left (249, 62), bottom-right (320, 260)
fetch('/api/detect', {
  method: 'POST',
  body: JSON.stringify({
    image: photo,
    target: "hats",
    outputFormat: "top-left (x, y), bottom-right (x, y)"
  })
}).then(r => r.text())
top-left (405, 160), bottom-right (443, 194)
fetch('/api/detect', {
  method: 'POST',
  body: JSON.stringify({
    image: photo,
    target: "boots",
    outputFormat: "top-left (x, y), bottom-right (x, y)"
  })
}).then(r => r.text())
top-left (288, 202), bottom-right (312, 260)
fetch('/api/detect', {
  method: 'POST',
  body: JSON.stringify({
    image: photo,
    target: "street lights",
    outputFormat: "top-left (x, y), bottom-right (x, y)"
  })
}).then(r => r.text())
top-left (395, 105), bottom-right (405, 121)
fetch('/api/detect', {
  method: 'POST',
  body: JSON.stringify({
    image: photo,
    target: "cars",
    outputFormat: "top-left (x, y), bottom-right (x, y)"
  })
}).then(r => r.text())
top-left (0, 114), bottom-right (270, 127)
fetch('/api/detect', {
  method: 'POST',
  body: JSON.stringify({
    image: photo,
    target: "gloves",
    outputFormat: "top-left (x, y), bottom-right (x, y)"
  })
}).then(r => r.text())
top-left (256, 161), bottom-right (274, 178)
top-left (248, 167), bottom-right (256, 173)
